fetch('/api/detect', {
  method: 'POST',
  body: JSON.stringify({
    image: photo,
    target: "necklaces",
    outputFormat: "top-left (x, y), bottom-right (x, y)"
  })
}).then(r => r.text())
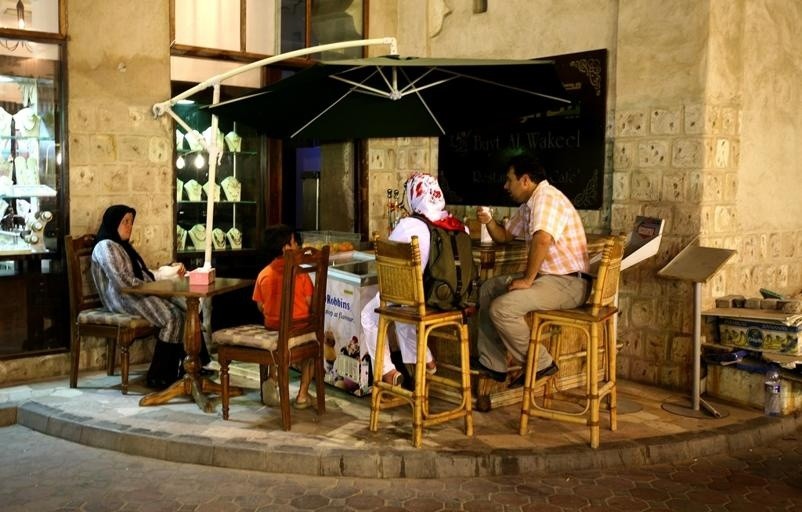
top-left (214, 234), bottom-right (226, 247)
top-left (228, 138), bottom-right (240, 152)
top-left (193, 232), bottom-right (207, 242)
top-left (226, 184), bottom-right (241, 201)
top-left (22, 119), bottom-right (37, 130)
top-left (177, 228), bottom-right (185, 245)
top-left (229, 232), bottom-right (243, 245)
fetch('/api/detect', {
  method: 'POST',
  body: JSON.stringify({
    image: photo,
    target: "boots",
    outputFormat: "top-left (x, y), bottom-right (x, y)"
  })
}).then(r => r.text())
top-left (148, 339), bottom-right (182, 388)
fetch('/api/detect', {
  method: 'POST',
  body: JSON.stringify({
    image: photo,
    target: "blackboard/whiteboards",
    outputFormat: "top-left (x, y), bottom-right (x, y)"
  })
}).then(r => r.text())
top-left (438, 49), bottom-right (607, 209)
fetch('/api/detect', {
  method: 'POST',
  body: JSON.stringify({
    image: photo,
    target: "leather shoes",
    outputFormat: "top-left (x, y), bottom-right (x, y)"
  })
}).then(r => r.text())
top-left (470, 356), bottom-right (506, 381)
top-left (508, 360), bottom-right (558, 388)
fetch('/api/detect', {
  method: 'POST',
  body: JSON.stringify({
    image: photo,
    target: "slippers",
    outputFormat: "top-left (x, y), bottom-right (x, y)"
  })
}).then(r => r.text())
top-left (262, 380), bottom-right (280, 405)
top-left (393, 371), bottom-right (403, 387)
top-left (294, 394), bottom-right (317, 408)
top-left (426, 366), bottom-right (436, 374)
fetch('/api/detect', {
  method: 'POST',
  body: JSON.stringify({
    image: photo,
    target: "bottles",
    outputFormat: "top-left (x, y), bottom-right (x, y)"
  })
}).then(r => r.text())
top-left (763, 364), bottom-right (782, 416)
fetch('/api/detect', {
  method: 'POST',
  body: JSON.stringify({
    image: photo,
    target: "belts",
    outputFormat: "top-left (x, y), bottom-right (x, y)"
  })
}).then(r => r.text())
top-left (568, 272), bottom-right (591, 280)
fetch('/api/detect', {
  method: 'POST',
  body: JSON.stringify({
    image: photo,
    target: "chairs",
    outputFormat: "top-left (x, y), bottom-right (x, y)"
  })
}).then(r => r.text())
top-left (365, 234), bottom-right (477, 447)
top-left (211, 242), bottom-right (332, 431)
top-left (64, 232), bottom-right (155, 397)
top-left (517, 230), bottom-right (627, 447)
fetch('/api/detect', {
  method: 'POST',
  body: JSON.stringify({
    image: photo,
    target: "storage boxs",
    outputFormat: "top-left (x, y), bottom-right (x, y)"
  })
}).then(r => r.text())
top-left (718, 318), bottom-right (802, 357)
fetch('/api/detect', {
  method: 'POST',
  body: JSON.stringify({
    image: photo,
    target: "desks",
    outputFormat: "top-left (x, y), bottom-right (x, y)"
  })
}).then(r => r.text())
top-left (422, 233), bottom-right (611, 410)
top-left (119, 269), bottom-right (255, 415)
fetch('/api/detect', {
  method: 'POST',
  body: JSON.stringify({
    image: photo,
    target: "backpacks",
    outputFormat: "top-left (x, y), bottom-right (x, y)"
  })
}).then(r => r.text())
top-left (410, 213), bottom-right (479, 325)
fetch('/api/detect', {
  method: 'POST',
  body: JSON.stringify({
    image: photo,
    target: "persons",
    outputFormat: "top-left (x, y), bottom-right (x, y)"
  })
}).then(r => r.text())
top-left (471, 158), bottom-right (593, 389)
top-left (252, 225), bottom-right (318, 410)
top-left (91, 205), bottom-right (215, 390)
top-left (361, 173), bottom-right (478, 396)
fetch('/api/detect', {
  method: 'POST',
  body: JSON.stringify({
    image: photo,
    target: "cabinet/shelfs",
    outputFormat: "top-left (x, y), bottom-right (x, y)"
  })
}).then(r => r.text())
top-left (176, 150), bottom-right (257, 255)
top-left (0, 132), bottom-right (57, 255)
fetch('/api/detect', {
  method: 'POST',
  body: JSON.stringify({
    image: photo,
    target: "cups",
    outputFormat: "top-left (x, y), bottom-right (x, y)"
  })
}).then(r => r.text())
top-left (481, 206), bottom-right (493, 222)
